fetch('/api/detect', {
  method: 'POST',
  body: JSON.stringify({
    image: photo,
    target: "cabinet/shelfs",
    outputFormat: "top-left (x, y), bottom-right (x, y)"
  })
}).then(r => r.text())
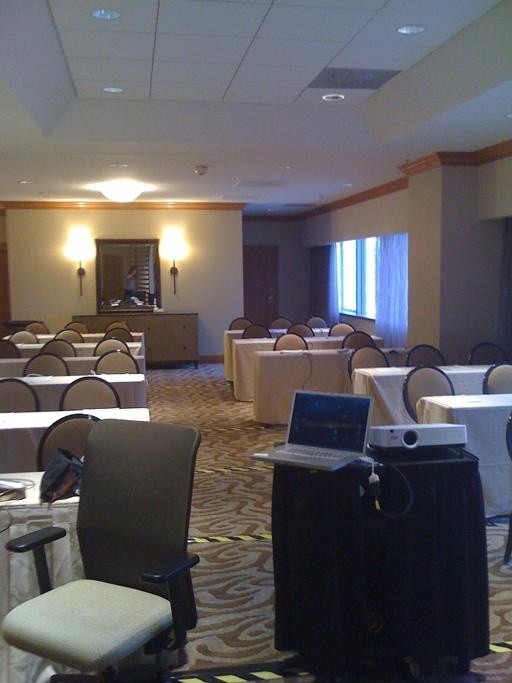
top-left (71, 312), bottom-right (199, 369)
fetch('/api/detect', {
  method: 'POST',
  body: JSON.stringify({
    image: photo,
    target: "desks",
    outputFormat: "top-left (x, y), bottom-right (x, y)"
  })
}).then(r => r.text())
top-left (0, 408), bottom-right (150, 474)
top-left (0, 470), bottom-right (85, 683)
top-left (415, 393), bottom-right (512, 519)
top-left (13, 342), bottom-right (141, 354)
top-left (253, 347), bottom-right (411, 423)
top-left (0, 373), bottom-right (146, 413)
top-left (224, 327), bottom-right (331, 382)
top-left (0, 355), bottom-right (146, 377)
top-left (232, 335), bottom-right (384, 401)
top-left (353, 365), bottom-right (512, 426)
top-left (2, 331), bottom-right (143, 344)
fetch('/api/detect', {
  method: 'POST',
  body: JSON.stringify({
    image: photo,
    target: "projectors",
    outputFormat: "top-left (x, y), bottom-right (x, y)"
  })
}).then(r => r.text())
top-left (370, 424), bottom-right (467, 449)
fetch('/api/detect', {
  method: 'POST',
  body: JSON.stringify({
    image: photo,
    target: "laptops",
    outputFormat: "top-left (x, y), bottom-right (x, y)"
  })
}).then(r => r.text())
top-left (251, 390), bottom-right (373, 471)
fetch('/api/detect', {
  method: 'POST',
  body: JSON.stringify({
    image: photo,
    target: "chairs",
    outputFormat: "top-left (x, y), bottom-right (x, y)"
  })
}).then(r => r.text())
top-left (94, 350), bottom-right (140, 375)
top-left (104, 327), bottom-right (134, 342)
top-left (287, 323), bottom-right (315, 337)
top-left (53, 328), bottom-right (84, 343)
top-left (342, 331), bottom-right (376, 349)
top-left (93, 338), bottom-right (130, 357)
top-left (268, 316), bottom-right (293, 329)
top-left (23, 321), bottom-right (50, 334)
top-left (482, 361), bottom-right (512, 394)
top-left (9, 329), bottom-right (39, 344)
top-left (59, 376), bottom-right (121, 411)
top-left (37, 413), bottom-right (101, 472)
top-left (229, 317), bottom-right (252, 330)
top-left (328, 322), bottom-right (356, 337)
top-left (63, 321), bottom-right (91, 334)
top-left (40, 339), bottom-right (77, 357)
top-left (403, 365), bottom-right (455, 425)
top-left (406, 344), bottom-right (447, 367)
top-left (469, 342), bottom-right (511, 365)
top-left (273, 332), bottom-right (309, 351)
top-left (348, 345), bottom-right (390, 383)
top-left (1, 419), bottom-right (202, 683)
top-left (307, 317), bottom-right (328, 328)
top-left (241, 323), bottom-right (272, 339)
top-left (0, 340), bottom-right (20, 359)
top-left (22, 352), bottom-right (70, 377)
top-left (0, 378), bottom-right (39, 413)
top-left (104, 320), bottom-right (132, 333)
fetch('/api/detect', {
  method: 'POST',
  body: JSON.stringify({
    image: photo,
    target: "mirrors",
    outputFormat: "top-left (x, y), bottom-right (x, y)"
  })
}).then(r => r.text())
top-left (94, 238), bottom-right (162, 314)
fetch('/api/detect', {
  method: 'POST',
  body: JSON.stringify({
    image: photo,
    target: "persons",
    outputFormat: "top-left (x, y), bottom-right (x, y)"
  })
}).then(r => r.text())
top-left (123, 266), bottom-right (139, 301)
